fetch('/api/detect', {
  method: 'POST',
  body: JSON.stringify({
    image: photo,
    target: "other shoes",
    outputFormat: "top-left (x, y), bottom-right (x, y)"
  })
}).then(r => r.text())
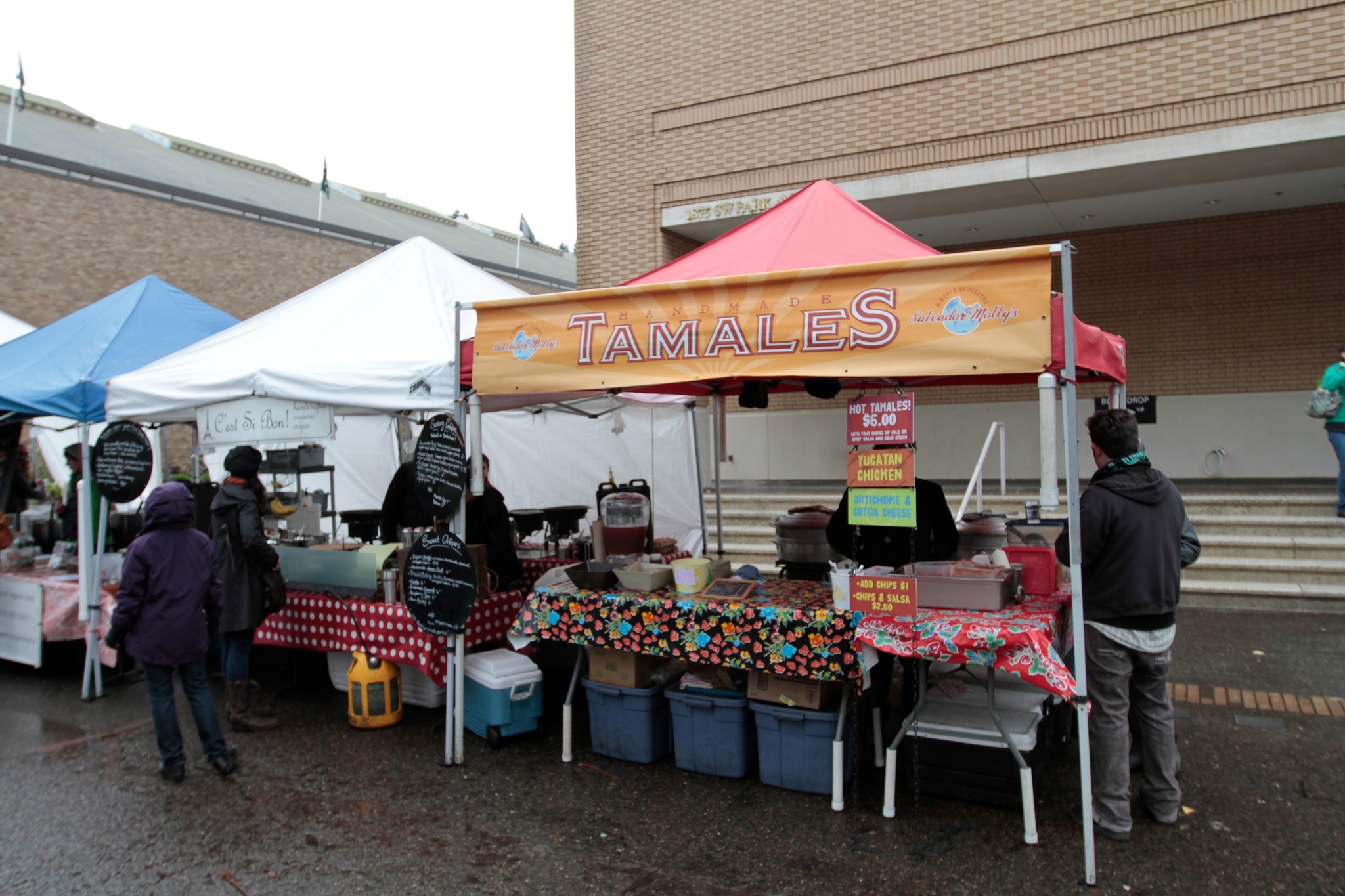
top-left (1129, 741), bottom-right (1144, 771)
top-left (1174, 742), bottom-right (1182, 776)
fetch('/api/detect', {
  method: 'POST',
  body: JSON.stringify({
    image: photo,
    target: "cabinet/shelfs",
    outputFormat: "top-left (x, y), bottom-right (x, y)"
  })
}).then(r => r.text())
top-left (256, 460), bottom-right (336, 542)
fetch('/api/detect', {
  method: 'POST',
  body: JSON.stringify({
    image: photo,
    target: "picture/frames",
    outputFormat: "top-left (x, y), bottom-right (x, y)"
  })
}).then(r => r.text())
top-left (699, 577), bottom-right (758, 601)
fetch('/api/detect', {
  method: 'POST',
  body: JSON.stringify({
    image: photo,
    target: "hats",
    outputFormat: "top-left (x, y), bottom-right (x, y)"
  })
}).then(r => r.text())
top-left (65, 444), bottom-right (94, 462)
top-left (17, 443), bottom-right (28, 457)
top-left (224, 445), bottom-right (262, 473)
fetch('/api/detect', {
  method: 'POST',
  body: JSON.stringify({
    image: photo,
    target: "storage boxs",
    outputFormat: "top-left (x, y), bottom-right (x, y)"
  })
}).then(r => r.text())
top-left (582, 641), bottom-right (849, 798)
top-left (265, 445), bottom-right (325, 469)
top-left (459, 645), bottom-right (544, 743)
top-left (322, 647), bottom-right (447, 710)
top-left (267, 488), bottom-right (329, 512)
top-left (1005, 519), bottom-right (1066, 564)
top-left (278, 529), bottom-right (329, 548)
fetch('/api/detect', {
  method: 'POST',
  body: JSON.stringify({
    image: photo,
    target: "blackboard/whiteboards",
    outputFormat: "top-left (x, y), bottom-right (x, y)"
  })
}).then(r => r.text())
top-left (400, 529), bottom-right (476, 638)
top-left (415, 413), bottom-right (467, 522)
top-left (91, 420), bottom-right (154, 504)
top-left (196, 395), bottom-right (337, 446)
top-left (0, 576), bottom-right (44, 669)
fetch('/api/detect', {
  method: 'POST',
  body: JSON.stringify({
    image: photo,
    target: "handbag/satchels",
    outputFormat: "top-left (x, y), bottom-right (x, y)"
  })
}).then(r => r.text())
top-left (219, 507), bottom-right (249, 572)
top-left (251, 566), bottom-right (287, 613)
top-left (1304, 385), bottom-right (1342, 418)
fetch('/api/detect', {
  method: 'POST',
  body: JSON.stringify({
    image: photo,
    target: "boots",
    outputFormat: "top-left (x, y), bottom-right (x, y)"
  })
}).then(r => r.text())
top-left (226, 681), bottom-right (273, 724)
top-left (227, 679), bottom-right (280, 732)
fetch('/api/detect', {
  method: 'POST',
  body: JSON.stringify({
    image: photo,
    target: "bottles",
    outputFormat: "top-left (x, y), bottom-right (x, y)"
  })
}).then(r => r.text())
top-left (403, 526), bottom-right (433, 549)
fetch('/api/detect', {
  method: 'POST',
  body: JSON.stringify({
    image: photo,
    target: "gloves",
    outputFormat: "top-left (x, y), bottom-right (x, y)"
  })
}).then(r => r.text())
top-left (104, 627), bottom-right (125, 649)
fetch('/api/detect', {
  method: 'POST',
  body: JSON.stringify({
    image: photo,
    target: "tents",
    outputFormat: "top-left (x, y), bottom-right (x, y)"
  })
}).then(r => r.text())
top-left (100, 235), bottom-right (708, 771)
top-left (0, 273), bottom-right (243, 702)
top-left (448, 182), bottom-right (1134, 888)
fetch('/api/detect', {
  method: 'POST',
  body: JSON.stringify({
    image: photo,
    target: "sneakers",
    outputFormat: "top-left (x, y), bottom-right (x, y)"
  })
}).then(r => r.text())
top-left (1140, 783), bottom-right (1176, 825)
top-left (1069, 802), bottom-right (1130, 842)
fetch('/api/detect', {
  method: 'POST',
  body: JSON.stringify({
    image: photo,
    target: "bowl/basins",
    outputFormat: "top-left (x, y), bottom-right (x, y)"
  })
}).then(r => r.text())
top-left (563, 559), bottom-right (673, 591)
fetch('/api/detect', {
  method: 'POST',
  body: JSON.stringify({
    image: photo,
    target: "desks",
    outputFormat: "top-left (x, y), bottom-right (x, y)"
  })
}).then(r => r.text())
top-left (251, 585), bottom-right (540, 768)
top-left (508, 571), bottom-right (885, 813)
top-left (1, 554), bottom-right (145, 680)
top-left (511, 540), bottom-right (692, 598)
top-left (854, 579), bottom-right (1078, 844)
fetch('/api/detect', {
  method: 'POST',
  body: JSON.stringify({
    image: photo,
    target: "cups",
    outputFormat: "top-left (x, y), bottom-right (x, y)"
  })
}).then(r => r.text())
top-left (381, 567), bottom-right (400, 603)
top-left (830, 568), bottom-right (865, 609)
top-left (670, 558), bottom-right (712, 592)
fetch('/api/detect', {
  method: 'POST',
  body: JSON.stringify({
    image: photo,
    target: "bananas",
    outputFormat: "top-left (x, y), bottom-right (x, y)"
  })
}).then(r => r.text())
top-left (270, 496), bottom-right (297, 515)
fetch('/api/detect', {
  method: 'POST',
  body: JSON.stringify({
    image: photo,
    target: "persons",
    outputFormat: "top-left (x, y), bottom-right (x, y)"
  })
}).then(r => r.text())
top-left (465, 451), bottom-right (494, 488)
top-left (1, 460), bottom-right (50, 531)
top-left (1056, 410), bottom-right (1187, 841)
top-left (51, 441), bottom-right (114, 555)
top-left (826, 433), bottom-right (962, 751)
top-left (104, 482), bottom-right (239, 782)
top-left (1318, 346), bottom-right (1345, 517)
top-left (379, 461), bottom-right (449, 544)
top-left (1120, 435), bottom-right (1202, 777)
top-left (209, 445), bottom-right (286, 731)
top-left (461, 476), bottom-right (522, 594)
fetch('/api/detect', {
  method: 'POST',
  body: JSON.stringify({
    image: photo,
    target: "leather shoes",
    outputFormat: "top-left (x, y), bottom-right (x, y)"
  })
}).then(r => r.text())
top-left (160, 769), bottom-right (184, 782)
top-left (213, 747), bottom-right (240, 775)
